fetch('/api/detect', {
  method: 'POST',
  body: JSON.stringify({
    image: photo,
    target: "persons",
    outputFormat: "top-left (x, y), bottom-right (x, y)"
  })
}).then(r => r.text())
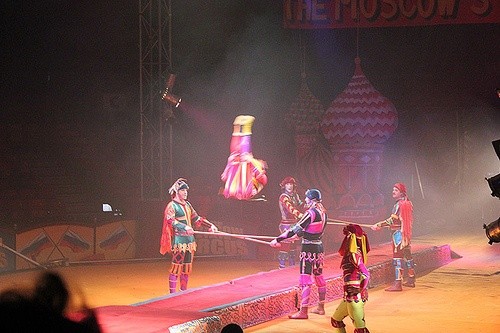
top-left (221, 115), bottom-right (266, 201)
top-left (270, 189), bottom-right (328, 319)
top-left (278, 177), bottom-right (308, 269)
top-left (331, 223), bottom-right (372, 333)
top-left (159, 178), bottom-right (218, 294)
top-left (371, 182), bottom-right (416, 292)
top-left (0, 266), bottom-right (104, 332)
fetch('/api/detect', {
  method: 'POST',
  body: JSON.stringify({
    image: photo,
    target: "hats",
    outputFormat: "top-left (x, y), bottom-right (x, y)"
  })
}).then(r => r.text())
top-left (282, 177), bottom-right (296, 186)
top-left (394, 183), bottom-right (406, 195)
top-left (168, 178), bottom-right (189, 195)
top-left (305, 189), bottom-right (321, 201)
